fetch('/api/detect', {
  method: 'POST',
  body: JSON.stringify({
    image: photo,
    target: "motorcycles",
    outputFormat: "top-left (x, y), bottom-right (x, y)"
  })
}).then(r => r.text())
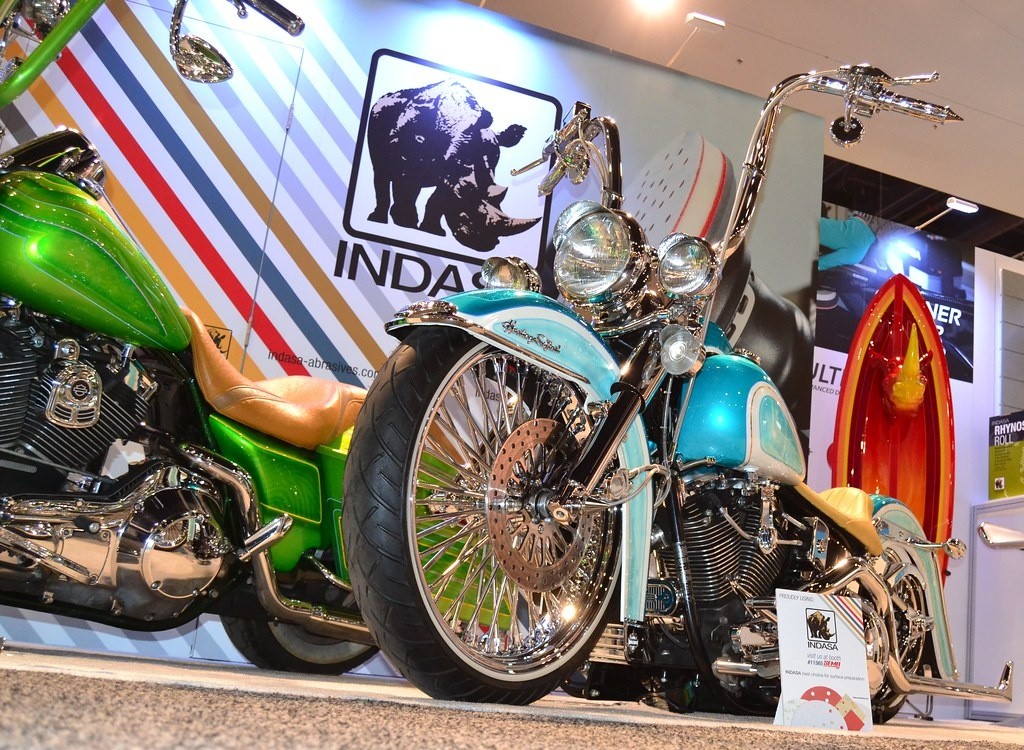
top-left (339, 65), bottom-right (1012, 726)
top-left (0, 1), bottom-right (381, 677)
